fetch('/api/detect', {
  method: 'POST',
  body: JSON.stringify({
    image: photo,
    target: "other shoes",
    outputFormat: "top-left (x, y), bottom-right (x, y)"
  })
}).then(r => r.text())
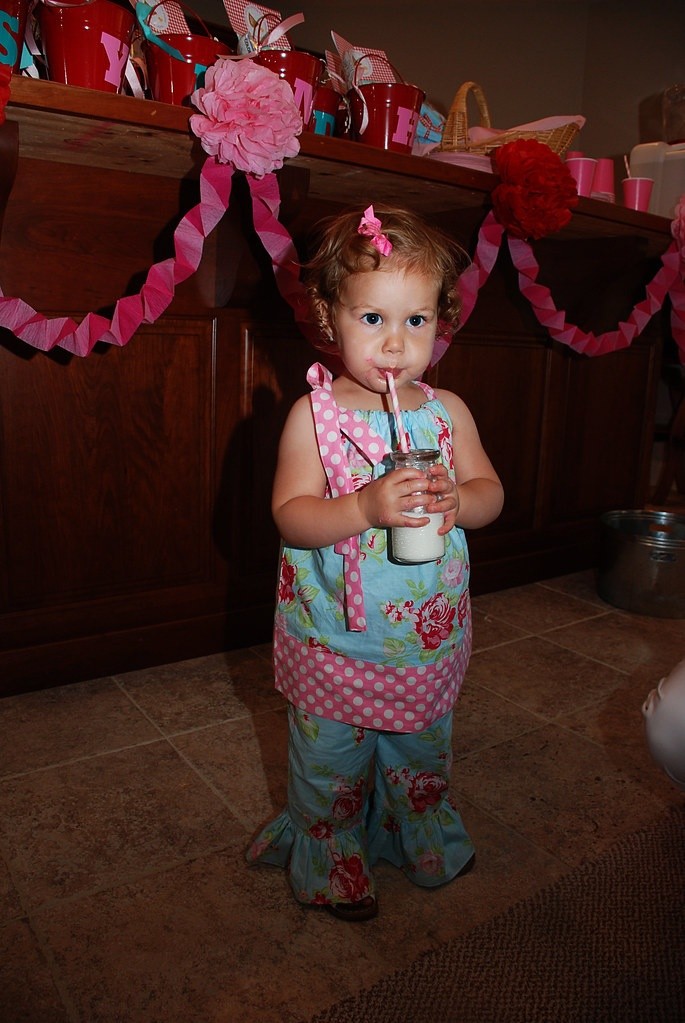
top-left (325, 894), bottom-right (378, 921)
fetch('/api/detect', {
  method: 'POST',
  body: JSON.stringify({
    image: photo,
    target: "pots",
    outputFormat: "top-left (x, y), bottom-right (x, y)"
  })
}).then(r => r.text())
top-left (597, 509), bottom-right (685, 617)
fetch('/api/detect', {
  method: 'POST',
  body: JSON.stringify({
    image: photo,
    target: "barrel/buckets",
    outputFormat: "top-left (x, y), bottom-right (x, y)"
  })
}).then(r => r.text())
top-left (345, 54), bottom-right (427, 156)
top-left (303, 83), bottom-right (343, 136)
top-left (1, 1), bottom-right (31, 74)
top-left (244, 14), bottom-right (328, 133)
top-left (38, 0), bottom-right (135, 96)
top-left (140, 1), bottom-right (235, 110)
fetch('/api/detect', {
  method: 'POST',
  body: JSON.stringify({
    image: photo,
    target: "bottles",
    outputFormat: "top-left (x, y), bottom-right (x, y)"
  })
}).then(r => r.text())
top-left (629, 141), bottom-right (685, 222)
top-left (390, 449), bottom-right (446, 566)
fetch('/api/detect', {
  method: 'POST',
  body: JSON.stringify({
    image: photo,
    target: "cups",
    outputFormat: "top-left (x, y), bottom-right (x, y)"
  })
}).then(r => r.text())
top-left (564, 151), bottom-right (617, 204)
top-left (621, 177), bottom-right (656, 212)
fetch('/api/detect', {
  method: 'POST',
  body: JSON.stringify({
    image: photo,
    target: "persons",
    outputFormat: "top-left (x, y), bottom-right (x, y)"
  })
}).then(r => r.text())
top-left (244, 203), bottom-right (506, 922)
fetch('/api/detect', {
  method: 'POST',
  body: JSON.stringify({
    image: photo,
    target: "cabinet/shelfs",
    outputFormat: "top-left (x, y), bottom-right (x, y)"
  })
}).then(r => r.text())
top-left (0, 70), bottom-right (681, 700)
